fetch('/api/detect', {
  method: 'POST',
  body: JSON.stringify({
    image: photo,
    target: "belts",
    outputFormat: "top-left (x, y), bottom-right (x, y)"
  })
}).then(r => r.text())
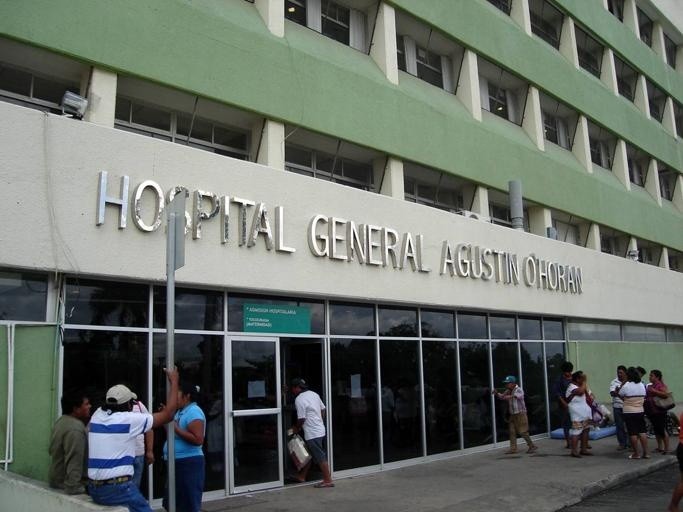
top-left (92, 477), bottom-right (128, 484)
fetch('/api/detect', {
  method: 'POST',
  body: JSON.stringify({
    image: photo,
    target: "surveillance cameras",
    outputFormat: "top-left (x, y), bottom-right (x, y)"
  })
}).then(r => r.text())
top-left (626, 250), bottom-right (638, 258)
top-left (60, 90), bottom-right (87, 115)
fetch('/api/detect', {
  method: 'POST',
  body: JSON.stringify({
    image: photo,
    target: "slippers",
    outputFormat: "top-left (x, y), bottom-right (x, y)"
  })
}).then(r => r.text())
top-left (314, 482), bottom-right (334, 488)
top-left (289, 473), bottom-right (305, 482)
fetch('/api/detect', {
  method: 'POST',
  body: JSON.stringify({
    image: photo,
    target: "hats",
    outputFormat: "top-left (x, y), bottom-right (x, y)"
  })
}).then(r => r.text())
top-left (106, 384), bottom-right (138, 404)
top-left (291, 378), bottom-right (307, 388)
top-left (503, 376), bottom-right (515, 382)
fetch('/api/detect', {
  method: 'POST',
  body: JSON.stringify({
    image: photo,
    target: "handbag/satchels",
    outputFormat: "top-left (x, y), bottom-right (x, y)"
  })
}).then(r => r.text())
top-left (655, 393), bottom-right (675, 410)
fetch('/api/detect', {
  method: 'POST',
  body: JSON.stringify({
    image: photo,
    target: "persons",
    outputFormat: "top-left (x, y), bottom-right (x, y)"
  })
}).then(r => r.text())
top-left (47, 385), bottom-right (91, 495)
top-left (550, 361), bottom-right (593, 449)
top-left (345, 374), bottom-right (490, 446)
top-left (131, 400), bottom-right (154, 489)
top-left (233, 403), bottom-right (247, 467)
top-left (610, 366), bottom-right (682, 511)
top-left (288, 378), bottom-right (333, 487)
top-left (157, 378), bottom-right (207, 510)
top-left (665, 410), bottom-right (682, 511)
top-left (564, 370), bottom-right (593, 458)
top-left (492, 375), bottom-right (537, 453)
top-left (85, 366), bottom-right (179, 512)
top-left (206, 394), bottom-right (236, 470)
